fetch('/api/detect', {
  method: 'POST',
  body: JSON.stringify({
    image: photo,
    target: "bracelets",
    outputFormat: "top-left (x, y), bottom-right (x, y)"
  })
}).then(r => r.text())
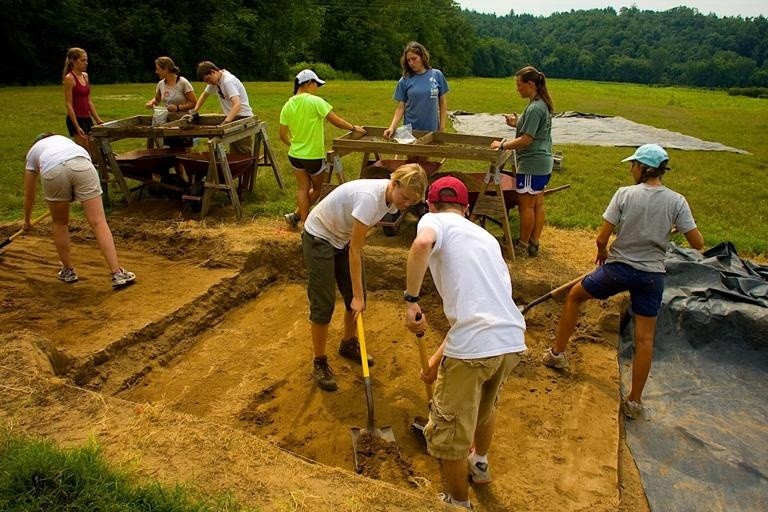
top-left (500, 142), bottom-right (504, 151)
top-left (176, 104), bottom-right (180, 112)
top-left (351, 125), bottom-right (356, 132)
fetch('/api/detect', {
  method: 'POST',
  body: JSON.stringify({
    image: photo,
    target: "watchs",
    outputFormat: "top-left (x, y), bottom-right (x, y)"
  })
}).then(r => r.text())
top-left (402, 288), bottom-right (421, 304)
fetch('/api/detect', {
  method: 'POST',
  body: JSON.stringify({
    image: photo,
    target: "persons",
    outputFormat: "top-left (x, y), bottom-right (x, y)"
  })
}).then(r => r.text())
top-left (540, 141), bottom-right (704, 421)
top-left (381, 40), bottom-right (451, 142)
top-left (145, 55), bottom-right (198, 185)
top-left (277, 68), bottom-right (369, 228)
top-left (22, 129), bottom-right (137, 288)
top-left (398, 174), bottom-right (529, 512)
top-left (193, 58), bottom-right (254, 194)
top-left (300, 162), bottom-right (429, 392)
top-left (62, 47), bottom-right (104, 154)
top-left (489, 65), bottom-right (555, 258)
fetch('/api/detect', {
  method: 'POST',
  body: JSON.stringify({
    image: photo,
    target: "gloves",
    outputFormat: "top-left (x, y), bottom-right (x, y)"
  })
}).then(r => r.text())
top-left (180, 109), bottom-right (197, 123)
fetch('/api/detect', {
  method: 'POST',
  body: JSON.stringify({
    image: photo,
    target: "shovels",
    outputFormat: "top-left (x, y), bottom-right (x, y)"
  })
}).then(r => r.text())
top-left (518, 219), bottom-right (698, 320)
top-left (411, 311), bottom-right (435, 433)
top-left (350, 311), bottom-right (401, 475)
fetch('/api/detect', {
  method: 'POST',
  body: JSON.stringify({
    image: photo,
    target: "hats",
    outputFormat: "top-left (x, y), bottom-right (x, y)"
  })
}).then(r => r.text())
top-left (620, 143), bottom-right (671, 169)
top-left (428, 175), bottom-right (469, 205)
top-left (296, 69), bottom-right (326, 86)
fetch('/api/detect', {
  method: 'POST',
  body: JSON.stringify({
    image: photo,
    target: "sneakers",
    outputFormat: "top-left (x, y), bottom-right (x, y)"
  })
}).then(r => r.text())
top-left (499, 238), bottom-right (539, 259)
top-left (467, 448), bottom-right (490, 485)
top-left (284, 212), bottom-right (299, 227)
top-left (311, 355), bottom-right (338, 391)
top-left (339, 336), bottom-right (375, 366)
top-left (111, 267), bottom-right (136, 286)
top-left (542, 347), bottom-right (570, 368)
top-left (623, 397), bottom-right (641, 420)
top-left (57, 268), bottom-right (79, 283)
